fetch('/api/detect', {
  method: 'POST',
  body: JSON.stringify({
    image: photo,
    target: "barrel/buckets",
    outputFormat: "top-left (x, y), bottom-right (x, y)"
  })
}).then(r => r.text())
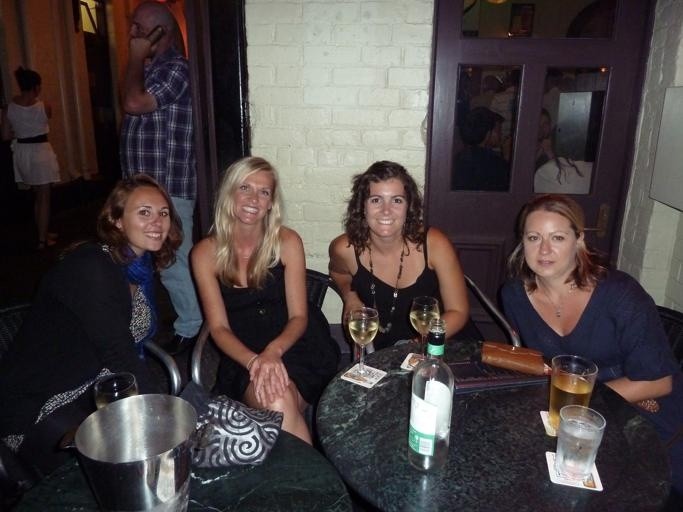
top-left (73, 393), bottom-right (199, 512)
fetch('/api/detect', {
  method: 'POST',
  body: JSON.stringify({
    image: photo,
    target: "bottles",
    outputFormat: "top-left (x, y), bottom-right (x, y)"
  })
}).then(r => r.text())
top-left (408, 318), bottom-right (456, 474)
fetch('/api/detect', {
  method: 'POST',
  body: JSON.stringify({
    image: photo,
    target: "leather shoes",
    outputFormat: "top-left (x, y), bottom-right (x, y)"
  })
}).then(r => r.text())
top-left (164, 332), bottom-right (200, 356)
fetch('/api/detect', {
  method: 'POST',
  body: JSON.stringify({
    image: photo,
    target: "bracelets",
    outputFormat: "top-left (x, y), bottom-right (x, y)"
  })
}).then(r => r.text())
top-left (245, 353), bottom-right (258, 369)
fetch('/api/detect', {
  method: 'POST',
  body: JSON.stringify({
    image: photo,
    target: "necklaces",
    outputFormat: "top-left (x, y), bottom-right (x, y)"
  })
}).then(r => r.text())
top-left (363, 239), bottom-right (408, 334)
top-left (537, 282), bottom-right (580, 319)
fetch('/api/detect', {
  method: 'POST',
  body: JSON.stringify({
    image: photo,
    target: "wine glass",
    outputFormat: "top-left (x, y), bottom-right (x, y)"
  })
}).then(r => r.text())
top-left (346, 294), bottom-right (441, 381)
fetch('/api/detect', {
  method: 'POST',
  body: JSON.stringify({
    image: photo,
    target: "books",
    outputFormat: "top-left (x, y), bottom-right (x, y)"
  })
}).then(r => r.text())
top-left (447, 360), bottom-right (553, 395)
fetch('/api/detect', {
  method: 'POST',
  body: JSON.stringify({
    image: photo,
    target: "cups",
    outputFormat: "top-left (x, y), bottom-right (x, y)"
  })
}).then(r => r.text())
top-left (547, 354), bottom-right (607, 484)
top-left (94, 372), bottom-right (138, 407)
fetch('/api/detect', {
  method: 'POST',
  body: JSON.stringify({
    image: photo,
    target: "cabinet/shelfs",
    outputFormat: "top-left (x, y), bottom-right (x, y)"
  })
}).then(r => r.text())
top-left (423, 3), bottom-right (656, 321)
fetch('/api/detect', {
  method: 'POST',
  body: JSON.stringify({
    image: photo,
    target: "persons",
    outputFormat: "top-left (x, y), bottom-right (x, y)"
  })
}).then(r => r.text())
top-left (187, 154), bottom-right (341, 448)
top-left (0, 172), bottom-right (188, 484)
top-left (450, 1), bottom-right (614, 195)
top-left (3, 66), bottom-right (61, 252)
top-left (115, 1), bottom-right (203, 357)
top-left (327, 159), bottom-right (483, 362)
top-left (498, 193), bottom-right (682, 500)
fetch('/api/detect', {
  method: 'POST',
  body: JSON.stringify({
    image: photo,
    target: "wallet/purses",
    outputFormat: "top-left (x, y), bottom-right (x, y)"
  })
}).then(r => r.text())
top-left (482, 341), bottom-right (545, 377)
